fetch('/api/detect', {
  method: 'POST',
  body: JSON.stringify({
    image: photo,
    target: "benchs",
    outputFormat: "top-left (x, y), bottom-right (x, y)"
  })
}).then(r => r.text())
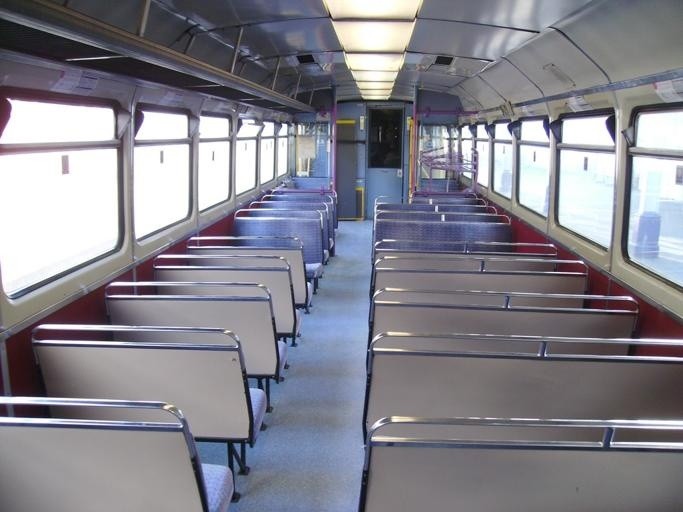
top-left (103, 278), bottom-right (290, 415)
top-left (359, 329), bottom-right (682, 447)
top-left (29, 323), bottom-right (267, 474)
top-left (367, 285), bottom-right (642, 374)
top-left (183, 234), bottom-right (315, 343)
top-left (358, 414), bottom-right (683, 512)
top-left (149, 250), bottom-right (303, 371)
top-left (0, 397), bottom-right (233, 507)
top-left (366, 191), bottom-right (592, 334)
top-left (230, 187), bottom-right (339, 295)
